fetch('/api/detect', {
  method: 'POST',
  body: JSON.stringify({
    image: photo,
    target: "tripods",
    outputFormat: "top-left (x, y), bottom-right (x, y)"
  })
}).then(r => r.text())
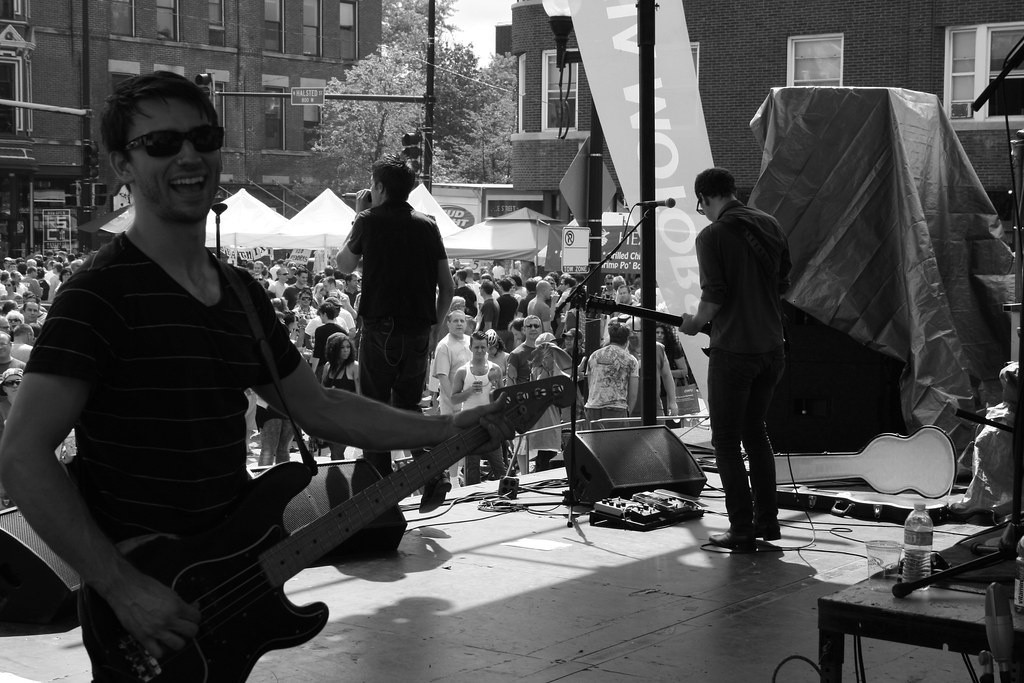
top-left (891, 44), bottom-right (1024, 599)
top-left (495, 208), bottom-right (655, 528)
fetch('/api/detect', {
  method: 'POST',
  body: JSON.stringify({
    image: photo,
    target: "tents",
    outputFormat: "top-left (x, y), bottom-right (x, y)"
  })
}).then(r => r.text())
top-left (78, 185), bottom-right (553, 269)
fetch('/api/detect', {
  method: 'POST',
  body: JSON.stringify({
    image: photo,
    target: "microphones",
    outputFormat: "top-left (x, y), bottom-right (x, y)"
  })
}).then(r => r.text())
top-left (636, 198), bottom-right (676, 209)
top-left (984, 581), bottom-right (1016, 683)
top-left (342, 192), bottom-right (372, 203)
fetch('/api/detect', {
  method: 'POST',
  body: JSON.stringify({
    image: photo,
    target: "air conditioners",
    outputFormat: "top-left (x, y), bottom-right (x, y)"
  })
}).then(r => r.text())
top-left (949, 103), bottom-right (973, 119)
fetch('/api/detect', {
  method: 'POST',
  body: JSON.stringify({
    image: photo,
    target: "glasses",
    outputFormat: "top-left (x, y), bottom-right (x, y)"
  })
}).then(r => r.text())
top-left (280, 273), bottom-right (288, 276)
top-left (2, 379), bottom-right (21, 388)
top-left (524, 324), bottom-right (541, 329)
top-left (8, 318), bottom-right (18, 323)
top-left (605, 282), bottom-right (613, 286)
top-left (255, 279), bottom-right (262, 281)
top-left (696, 199), bottom-right (705, 215)
top-left (119, 124), bottom-right (224, 157)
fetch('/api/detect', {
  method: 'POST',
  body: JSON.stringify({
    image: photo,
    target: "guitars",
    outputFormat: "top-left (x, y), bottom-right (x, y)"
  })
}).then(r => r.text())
top-left (585, 290), bottom-right (790, 373)
top-left (78, 374), bottom-right (580, 679)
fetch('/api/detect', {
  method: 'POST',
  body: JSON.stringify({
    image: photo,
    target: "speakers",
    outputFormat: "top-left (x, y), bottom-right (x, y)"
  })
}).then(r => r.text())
top-left (250, 456), bottom-right (407, 568)
top-left (0, 504), bottom-right (82, 624)
top-left (563, 425), bottom-right (708, 507)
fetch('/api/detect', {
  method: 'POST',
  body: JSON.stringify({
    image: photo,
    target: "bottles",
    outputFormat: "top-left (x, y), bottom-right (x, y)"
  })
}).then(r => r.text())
top-left (1014, 555), bottom-right (1024, 615)
top-left (903, 502), bottom-right (934, 590)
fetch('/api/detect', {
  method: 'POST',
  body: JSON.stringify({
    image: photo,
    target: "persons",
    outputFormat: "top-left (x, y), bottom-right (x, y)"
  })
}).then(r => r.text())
top-left (0, 248), bottom-right (690, 513)
top-left (0, 70), bottom-right (517, 683)
top-left (336, 159), bottom-right (455, 513)
top-left (679, 168), bottom-right (792, 552)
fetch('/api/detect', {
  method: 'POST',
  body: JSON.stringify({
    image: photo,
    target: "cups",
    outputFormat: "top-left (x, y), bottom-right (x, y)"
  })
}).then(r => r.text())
top-left (865, 541), bottom-right (902, 593)
top-left (473, 381), bottom-right (483, 394)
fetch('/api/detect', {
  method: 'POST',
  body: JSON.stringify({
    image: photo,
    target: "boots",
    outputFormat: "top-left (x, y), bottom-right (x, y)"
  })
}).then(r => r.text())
top-left (413, 448), bottom-right (452, 512)
top-left (362, 449), bottom-right (393, 478)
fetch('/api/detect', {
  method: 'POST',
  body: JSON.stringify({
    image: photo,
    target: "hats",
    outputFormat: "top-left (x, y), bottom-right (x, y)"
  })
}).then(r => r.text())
top-left (1, 367), bottom-right (24, 380)
top-left (535, 331), bottom-right (556, 346)
top-left (562, 328), bottom-right (583, 341)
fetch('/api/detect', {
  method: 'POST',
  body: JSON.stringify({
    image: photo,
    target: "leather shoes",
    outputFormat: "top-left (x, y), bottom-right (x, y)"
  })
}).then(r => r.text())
top-left (709, 530), bottom-right (758, 554)
top-left (753, 523), bottom-right (782, 541)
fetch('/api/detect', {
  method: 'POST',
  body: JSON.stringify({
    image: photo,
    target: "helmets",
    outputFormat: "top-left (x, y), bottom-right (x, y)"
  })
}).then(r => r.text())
top-left (486, 329), bottom-right (498, 347)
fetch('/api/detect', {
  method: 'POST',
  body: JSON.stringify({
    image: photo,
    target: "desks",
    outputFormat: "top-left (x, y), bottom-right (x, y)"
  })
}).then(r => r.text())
top-left (816, 521), bottom-right (1024, 683)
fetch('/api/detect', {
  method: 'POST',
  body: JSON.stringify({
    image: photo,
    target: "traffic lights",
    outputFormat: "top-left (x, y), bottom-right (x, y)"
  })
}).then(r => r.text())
top-left (401, 132), bottom-right (421, 174)
top-left (196, 71), bottom-right (215, 97)
top-left (84, 143), bottom-right (100, 181)
